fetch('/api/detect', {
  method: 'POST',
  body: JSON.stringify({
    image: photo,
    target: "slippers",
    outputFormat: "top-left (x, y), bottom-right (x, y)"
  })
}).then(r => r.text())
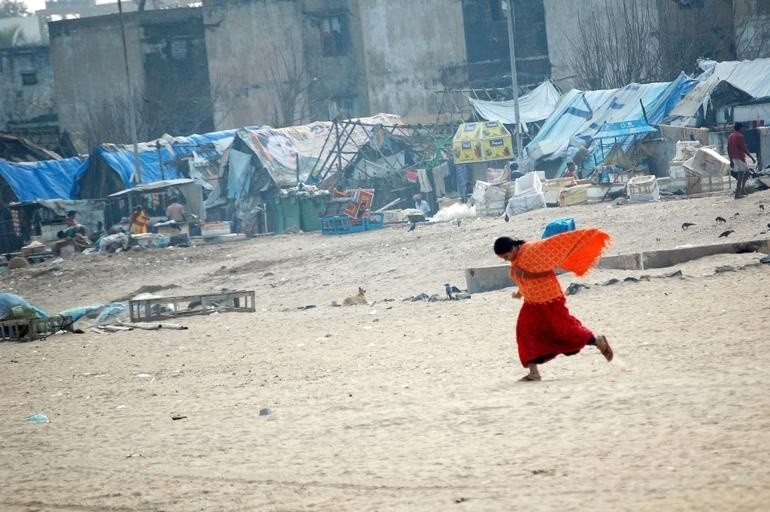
top-left (600, 336), bottom-right (612, 361)
top-left (518, 375), bottom-right (542, 381)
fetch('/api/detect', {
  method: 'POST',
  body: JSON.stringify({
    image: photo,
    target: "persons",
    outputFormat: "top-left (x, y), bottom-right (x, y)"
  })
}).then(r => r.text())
top-left (132, 204), bottom-right (150, 233)
top-left (727, 121), bottom-right (756, 199)
top-left (565, 161), bottom-right (577, 180)
top-left (202, 186), bottom-right (209, 200)
top-left (509, 162), bottom-right (524, 181)
top-left (110, 216), bottom-right (125, 234)
top-left (153, 198), bottom-right (165, 217)
top-left (413, 193), bottom-right (431, 218)
top-left (494, 228), bottom-right (613, 382)
top-left (30, 219), bottom-right (37, 235)
top-left (258, 129), bottom-right (320, 178)
top-left (166, 196), bottom-right (185, 222)
top-left (56, 210), bottom-right (105, 239)
top-left (487, 168), bottom-right (496, 182)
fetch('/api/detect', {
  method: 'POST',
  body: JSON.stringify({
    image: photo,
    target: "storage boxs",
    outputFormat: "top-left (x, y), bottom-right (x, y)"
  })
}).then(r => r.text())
top-left (471, 170), bottom-right (587, 217)
top-left (682, 145), bottom-right (730, 195)
top-left (628, 176), bottom-right (658, 201)
top-left (200, 223), bottom-right (231, 236)
